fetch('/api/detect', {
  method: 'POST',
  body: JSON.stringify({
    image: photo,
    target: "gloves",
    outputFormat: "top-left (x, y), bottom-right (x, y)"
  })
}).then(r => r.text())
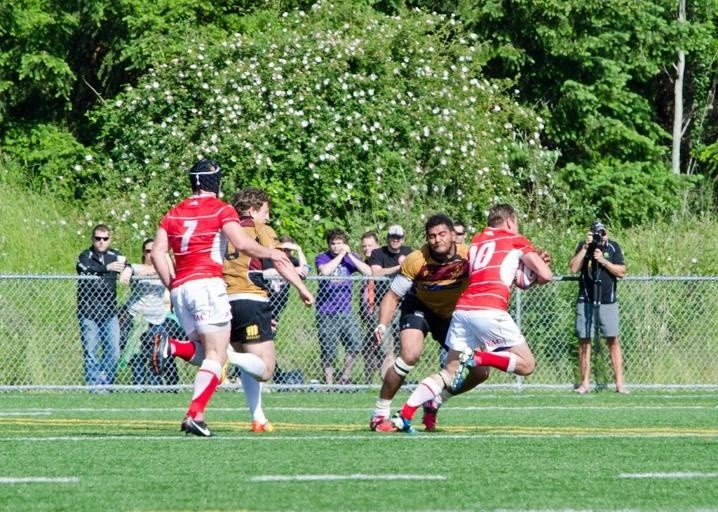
top-left (373, 324), bottom-right (387, 343)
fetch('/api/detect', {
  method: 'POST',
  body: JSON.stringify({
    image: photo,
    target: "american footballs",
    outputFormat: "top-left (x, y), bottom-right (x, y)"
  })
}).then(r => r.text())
top-left (514, 259), bottom-right (538, 289)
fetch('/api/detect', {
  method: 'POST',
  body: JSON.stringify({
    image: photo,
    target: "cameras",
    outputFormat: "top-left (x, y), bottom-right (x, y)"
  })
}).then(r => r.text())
top-left (592, 231), bottom-right (603, 243)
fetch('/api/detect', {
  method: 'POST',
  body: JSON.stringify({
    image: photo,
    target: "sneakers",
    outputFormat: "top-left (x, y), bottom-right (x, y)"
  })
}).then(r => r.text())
top-left (449, 344), bottom-right (479, 392)
top-left (150, 331), bottom-right (172, 376)
top-left (423, 398), bottom-right (436, 431)
top-left (390, 410), bottom-right (417, 433)
top-left (181, 414), bottom-right (218, 439)
top-left (250, 420), bottom-right (274, 435)
top-left (366, 415), bottom-right (399, 433)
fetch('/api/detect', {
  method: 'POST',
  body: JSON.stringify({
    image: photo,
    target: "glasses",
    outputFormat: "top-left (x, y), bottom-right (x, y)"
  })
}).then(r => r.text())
top-left (456, 233), bottom-right (465, 236)
top-left (94, 237), bottom-right (110, 241)
top-left (144, 249), bottom-right (151, 253)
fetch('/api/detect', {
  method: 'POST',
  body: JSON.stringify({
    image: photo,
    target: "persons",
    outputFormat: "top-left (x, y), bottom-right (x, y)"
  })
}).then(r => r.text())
top-left (570, 218), bottom-right (628, 395)
top-left (224, 188), bottom-right (315, 433)
top-left (119, 238), bottom-right (168, 392)
top-left (314, 228), bottom-right (372, 385)
top-left (358, 230), bottom-right (381, 384)
top-left (153, 159), bottom-right (291, 436)
top-left (391, 203), bottom-right (555, 434)
top-left (436, 220), bottom-right (468, 368)
top-left (370, 224), bottom-right (415, 385)
top-left (369, 212), bottom-right (489, 433)
top-left (76, 225), bottom-right (127, 393)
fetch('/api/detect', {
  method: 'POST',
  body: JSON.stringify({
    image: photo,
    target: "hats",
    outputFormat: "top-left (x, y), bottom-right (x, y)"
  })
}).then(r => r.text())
top-left (591, 219), bottom-right (607, 234)
top-left (388, 225), bottom-right (404, 240)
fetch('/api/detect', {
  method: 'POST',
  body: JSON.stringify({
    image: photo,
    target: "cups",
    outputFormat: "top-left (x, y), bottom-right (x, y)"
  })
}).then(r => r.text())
top-left (300, 264), bottom-right (313, 279)
top-left (117, 254), bottom-right (126, 264)
top-left (294, 379), bottom-right (303, 392)
top-left (310, 379), bottom-right (319, 392)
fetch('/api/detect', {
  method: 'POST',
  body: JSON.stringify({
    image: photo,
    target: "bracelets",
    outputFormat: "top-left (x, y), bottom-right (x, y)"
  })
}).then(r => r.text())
top-left (374, 324), bottom-right (386, 333)
